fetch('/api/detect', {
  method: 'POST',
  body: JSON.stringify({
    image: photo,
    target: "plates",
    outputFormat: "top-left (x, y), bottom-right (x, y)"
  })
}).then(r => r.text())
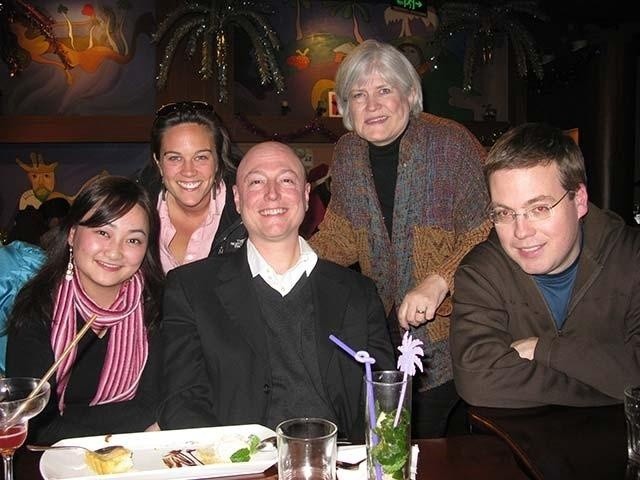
top-left (40, 423), bottom-right (288, 480)
top-left (336, 444), bottom-right (369, 480)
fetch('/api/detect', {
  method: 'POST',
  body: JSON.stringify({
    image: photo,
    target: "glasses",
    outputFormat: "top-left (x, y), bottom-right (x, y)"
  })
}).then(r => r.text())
top-left (154, 99), bottom-right (213, 117)
top-left (486, 204), bottom-right (559, 222)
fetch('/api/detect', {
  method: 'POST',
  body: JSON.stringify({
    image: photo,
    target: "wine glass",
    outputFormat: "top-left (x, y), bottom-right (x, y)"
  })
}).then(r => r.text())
top-left (0, 374), bottom-right (51, 480)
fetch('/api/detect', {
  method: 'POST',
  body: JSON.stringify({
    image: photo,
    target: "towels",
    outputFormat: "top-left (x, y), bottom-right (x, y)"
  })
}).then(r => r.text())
top-left (335, 443), bottom-right (420, 480)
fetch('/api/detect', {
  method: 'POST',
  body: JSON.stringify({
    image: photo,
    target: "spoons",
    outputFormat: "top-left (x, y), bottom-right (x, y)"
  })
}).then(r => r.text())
top-left (26, 443), bottom-right (123, 456)
top-left (336, 458), bottom-right (366, 472)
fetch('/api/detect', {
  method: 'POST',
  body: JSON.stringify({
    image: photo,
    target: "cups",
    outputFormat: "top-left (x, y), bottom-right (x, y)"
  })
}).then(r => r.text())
top-left (276, 417), bottom-right (338, 480)
top-left (365, 370), bottom-right (412, 480)
top-left (623, 384), bottom-right (640, 466)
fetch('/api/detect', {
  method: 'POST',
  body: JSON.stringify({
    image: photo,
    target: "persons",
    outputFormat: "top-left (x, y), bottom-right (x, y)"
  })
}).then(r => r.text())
top-left (307, 40), bottom-right (494, 438)
top-left (450, 121), bottom-right (640, 408)
top-left (145, 100), bottom-right (249, 276)
top-left (156, 142), bottom-right (399, 439)
top-left (6, 175), bottom-right (165, 445)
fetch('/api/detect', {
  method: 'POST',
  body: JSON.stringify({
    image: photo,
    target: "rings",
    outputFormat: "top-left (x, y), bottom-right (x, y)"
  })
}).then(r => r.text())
top-left (418, 310), bottom-right (425, 314)
